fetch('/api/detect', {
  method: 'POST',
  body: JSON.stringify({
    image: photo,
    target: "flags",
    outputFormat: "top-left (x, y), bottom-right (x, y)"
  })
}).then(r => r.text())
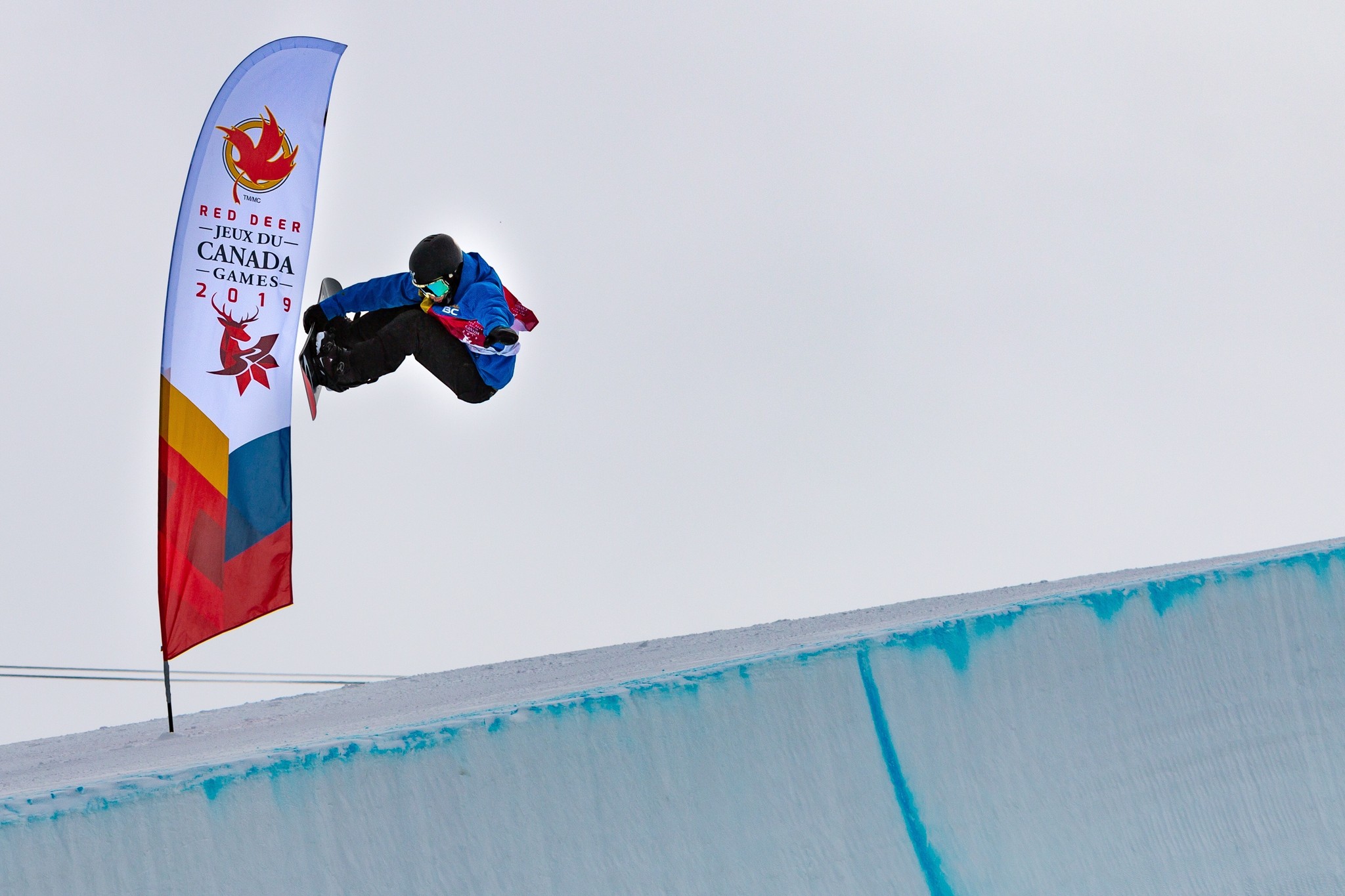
top-left (158, 36), bottom-right (347, 662)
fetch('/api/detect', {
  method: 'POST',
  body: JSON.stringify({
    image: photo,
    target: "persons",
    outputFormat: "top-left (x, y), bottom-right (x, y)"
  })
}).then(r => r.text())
top-left (303, 233), bottom-right (520, 404)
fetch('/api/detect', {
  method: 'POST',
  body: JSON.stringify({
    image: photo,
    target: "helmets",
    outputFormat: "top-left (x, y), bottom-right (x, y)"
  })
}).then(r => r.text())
top-left (409, 234), bottom-right (463, 307)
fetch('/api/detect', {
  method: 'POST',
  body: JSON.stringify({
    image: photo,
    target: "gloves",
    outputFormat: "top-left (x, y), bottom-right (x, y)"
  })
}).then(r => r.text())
top-left (483, 327), bottom-right (518, 349)
top-left (303, 304), bottom-right (327, 334)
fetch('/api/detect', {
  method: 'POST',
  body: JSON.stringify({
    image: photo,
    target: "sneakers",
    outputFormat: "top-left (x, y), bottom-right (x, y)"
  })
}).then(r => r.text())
top-left (315, 331), bottom-right (349, 392)
top-left (330, 315), bottom-right (351, 350)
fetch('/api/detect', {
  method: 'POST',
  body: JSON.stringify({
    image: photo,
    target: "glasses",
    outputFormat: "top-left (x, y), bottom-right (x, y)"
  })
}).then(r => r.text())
top-left (419, 280), bottom-right (448, 298)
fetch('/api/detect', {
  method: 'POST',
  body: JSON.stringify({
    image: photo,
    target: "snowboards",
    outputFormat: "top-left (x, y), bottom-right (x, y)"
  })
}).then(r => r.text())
top-left (298, 275), bottom-right (345, 423)
